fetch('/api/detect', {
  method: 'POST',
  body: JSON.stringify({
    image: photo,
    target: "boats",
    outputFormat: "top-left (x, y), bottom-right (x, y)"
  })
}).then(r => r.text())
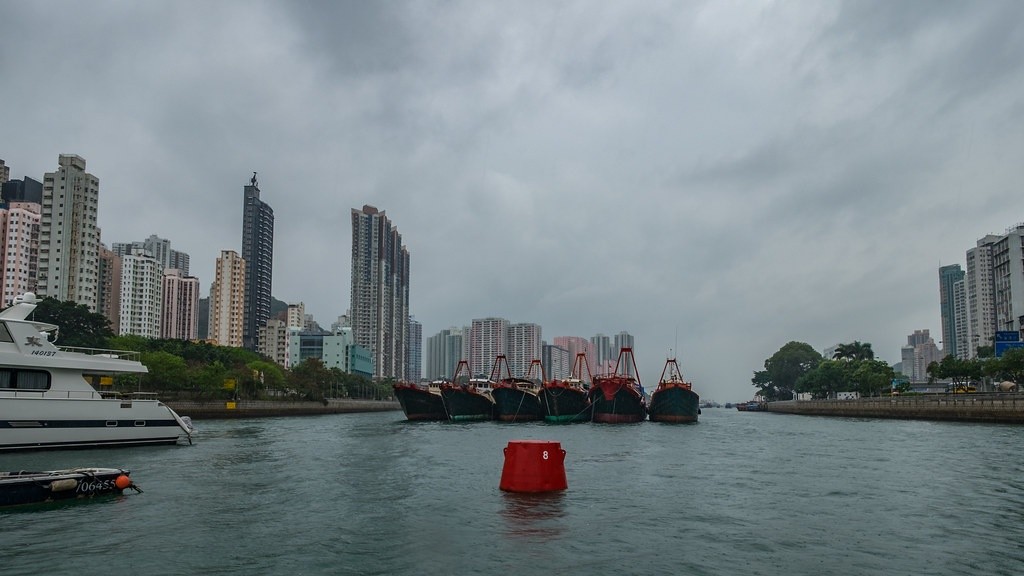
top-left (534, 353), bottom-right (594, 425)
top-left (490, 359), bottom-right (546, 422)
top-left (725, 403), bottom-right (732, 408)
top-left (587, 346), bottom-right (648, 424)
top-left (439, 353), bottom-right (511, 423)
top-left (0, 468), bottom-right (144, 504)
top-left (649, 349), bottom-right (701, 425)
top-left (0, 294), bottom-right (196, 452)
top-left (393, 360), bottom-right (473, 421)
top-left (735, 395), bottom-right (768, 412)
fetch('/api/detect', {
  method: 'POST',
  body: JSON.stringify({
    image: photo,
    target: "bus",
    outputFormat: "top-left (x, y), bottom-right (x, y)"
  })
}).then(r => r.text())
top-left (988, 377), bottom-right (1003, 386)
top-left (944, 382), bottom-right (976, 393)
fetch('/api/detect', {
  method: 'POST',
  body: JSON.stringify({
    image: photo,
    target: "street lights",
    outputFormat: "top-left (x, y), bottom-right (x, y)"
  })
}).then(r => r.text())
top-left (902, 356), bottom-right (914, 382)
top-left (939, 340), bottom-right (946, 357)
top-left (963, 334), bottom-right (981, 360)
top-left (919, 357), bottom-right (928, 382)
top-left (867, 356), bottom-right (879, 399)
